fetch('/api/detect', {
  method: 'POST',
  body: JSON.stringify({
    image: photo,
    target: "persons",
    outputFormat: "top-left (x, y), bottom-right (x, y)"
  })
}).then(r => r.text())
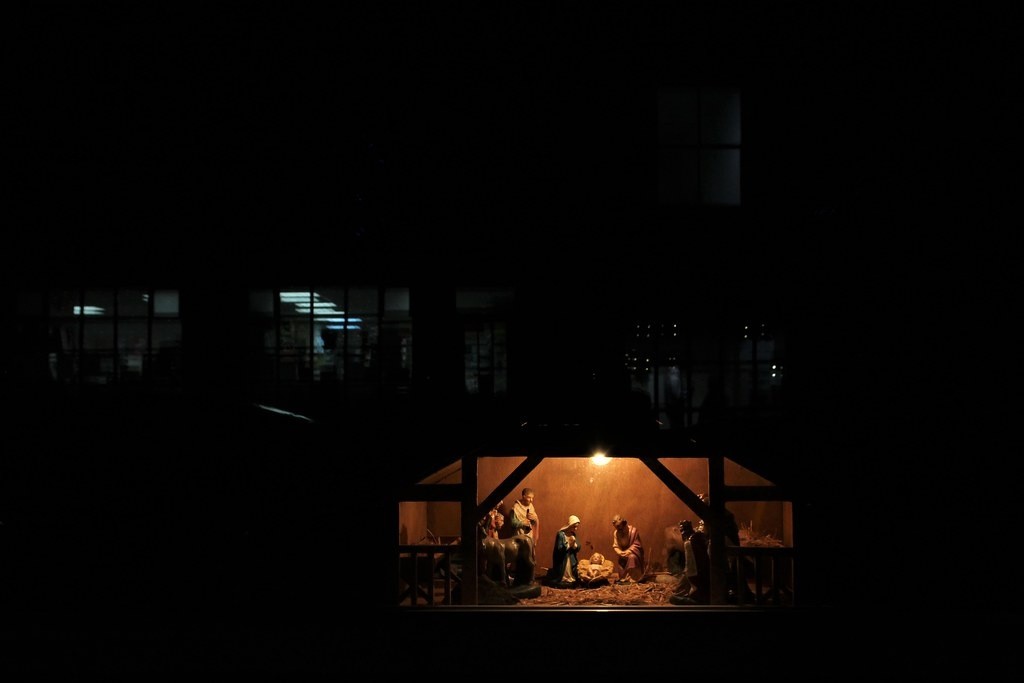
top-left (535, 515), bottom-right (581, 588)
top-left (670, 493), bottom-right (741, 605)
top-left (611, 514), bottom-right (648, 584)
top-left (508, 488), bottom-right (539, 584)
top-left (587, 552), bottom-right (605, 579)
top-left (482, 512), bottom-right (515, 587)
